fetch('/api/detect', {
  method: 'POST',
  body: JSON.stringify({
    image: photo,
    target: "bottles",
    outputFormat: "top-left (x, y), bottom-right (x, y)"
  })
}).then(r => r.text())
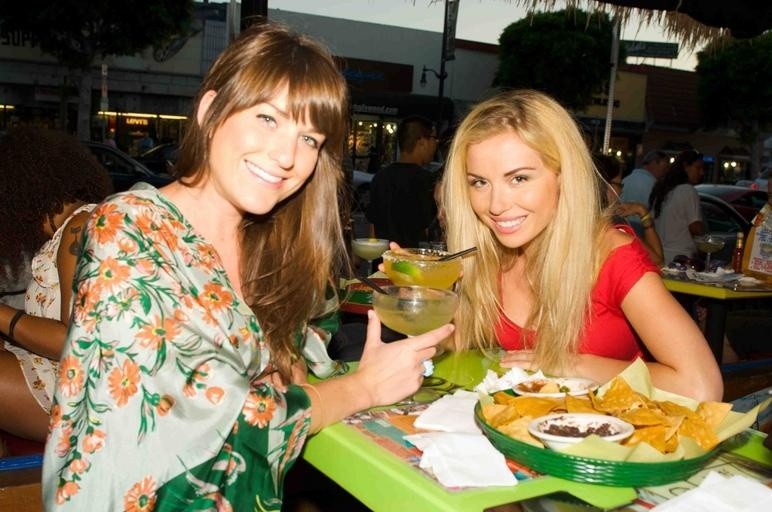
top-left (732, 232), bottom-right (745, 273)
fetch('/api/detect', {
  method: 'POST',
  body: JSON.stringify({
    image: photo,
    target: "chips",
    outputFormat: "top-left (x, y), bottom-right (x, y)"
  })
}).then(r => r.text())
top-left (493, 376), bottom-right (735, 453)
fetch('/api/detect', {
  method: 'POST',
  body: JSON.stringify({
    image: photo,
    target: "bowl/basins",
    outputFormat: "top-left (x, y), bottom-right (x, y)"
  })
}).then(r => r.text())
top-left (528, 408), bottom-right (635, 455)
top-left (511, 375), bottom-right (600, 404)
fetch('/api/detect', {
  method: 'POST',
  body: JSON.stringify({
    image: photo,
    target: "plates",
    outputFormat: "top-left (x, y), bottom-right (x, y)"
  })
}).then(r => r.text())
top-left (461, 398), bottom-right (733, 487)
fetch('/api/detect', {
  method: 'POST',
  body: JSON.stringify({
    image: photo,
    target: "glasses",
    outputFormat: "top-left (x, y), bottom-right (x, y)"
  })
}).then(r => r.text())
top-left (612, 181), bottom-right (624, 189)
top-left (673, 254), bottom-right (705, 272)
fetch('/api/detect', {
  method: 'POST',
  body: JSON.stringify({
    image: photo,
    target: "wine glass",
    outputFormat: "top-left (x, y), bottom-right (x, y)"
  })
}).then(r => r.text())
top-left (373, 284), bottom-right (460, 411)
top-left (351, 237), bottom-right (389, 281)
top-left (692, 231), bottom-right (726, 278)
top-left (382, 247), bottom-right (462, 392)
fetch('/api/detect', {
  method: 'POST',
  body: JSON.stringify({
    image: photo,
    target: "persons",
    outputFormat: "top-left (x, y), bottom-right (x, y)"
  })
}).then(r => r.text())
top-left (138, 132), bottom-right (154, 152)
top-left (103, 132), bottom-right (116, 148)
top-left (367, 85), bottom-right (725, 413)
top-left (0, 123), bottom-right (114, 443)
top-left (595, 148), bottom-right (704, 267)
top-left (364, 114), bottom-right (446, 274)
top-left (42, 13), bottom-right (457, 512)
